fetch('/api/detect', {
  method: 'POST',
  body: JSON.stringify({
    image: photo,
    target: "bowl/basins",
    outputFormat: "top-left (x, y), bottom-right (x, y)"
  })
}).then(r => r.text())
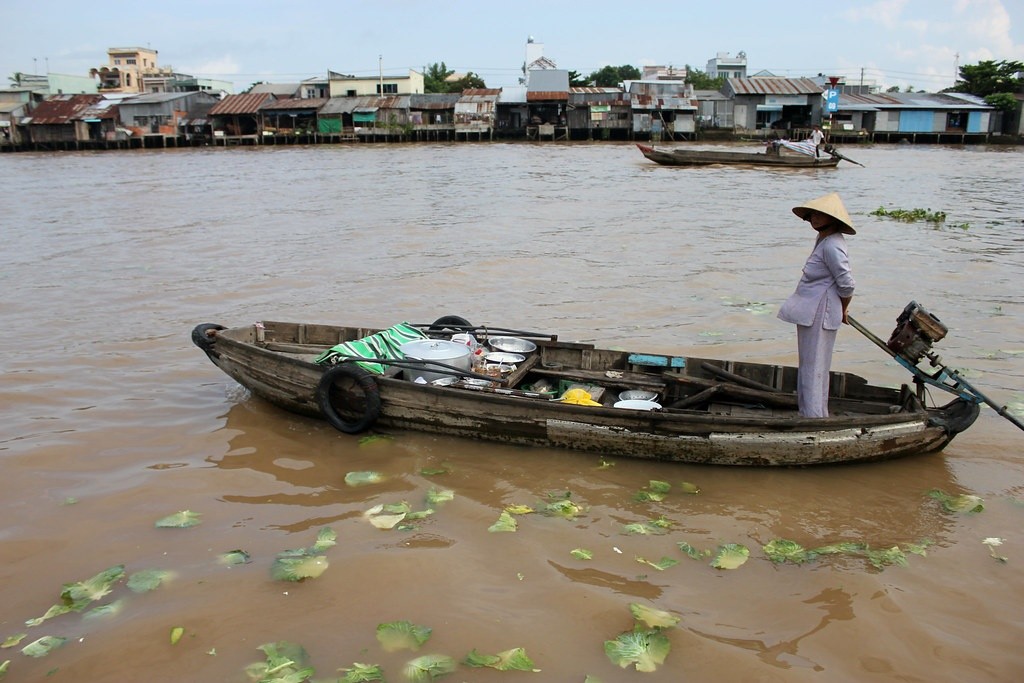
top-left (619, 390), bottom-right (658, 401)
top-left (486, 363), bottom-right (511, 379)
top-left (522, 384), bottom-right (559, 395)
top-left (488, 336), bottom-right (537, 359)
top-left (485, 352), bottom-right (526, 367)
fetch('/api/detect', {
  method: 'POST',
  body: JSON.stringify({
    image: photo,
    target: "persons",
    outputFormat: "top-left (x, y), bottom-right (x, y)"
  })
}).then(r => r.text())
top-left (777, 193), bottom-right (856, 419)
top-left (808, 125), bottom-right (827, 158)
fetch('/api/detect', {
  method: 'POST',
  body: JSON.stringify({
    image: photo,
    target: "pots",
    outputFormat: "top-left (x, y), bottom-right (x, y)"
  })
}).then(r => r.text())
top-left (401, 340), bottom-right (471, 385)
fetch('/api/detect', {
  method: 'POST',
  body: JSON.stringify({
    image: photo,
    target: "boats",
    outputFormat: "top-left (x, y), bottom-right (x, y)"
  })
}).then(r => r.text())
top-left (635, 142), bottom-right (842, 168)
top-left (191, 319), bottom-right (983, 466)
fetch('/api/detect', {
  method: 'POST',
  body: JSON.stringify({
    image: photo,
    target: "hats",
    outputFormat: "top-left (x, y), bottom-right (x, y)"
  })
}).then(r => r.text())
top-left (792, 192), bottom-right (856, 235)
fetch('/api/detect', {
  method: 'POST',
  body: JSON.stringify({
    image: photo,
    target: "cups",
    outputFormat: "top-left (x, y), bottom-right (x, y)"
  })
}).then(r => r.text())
top-left (488, 367), bottom-right (501, 387)
top-left (475, 362), bottom-right (487, 374)
